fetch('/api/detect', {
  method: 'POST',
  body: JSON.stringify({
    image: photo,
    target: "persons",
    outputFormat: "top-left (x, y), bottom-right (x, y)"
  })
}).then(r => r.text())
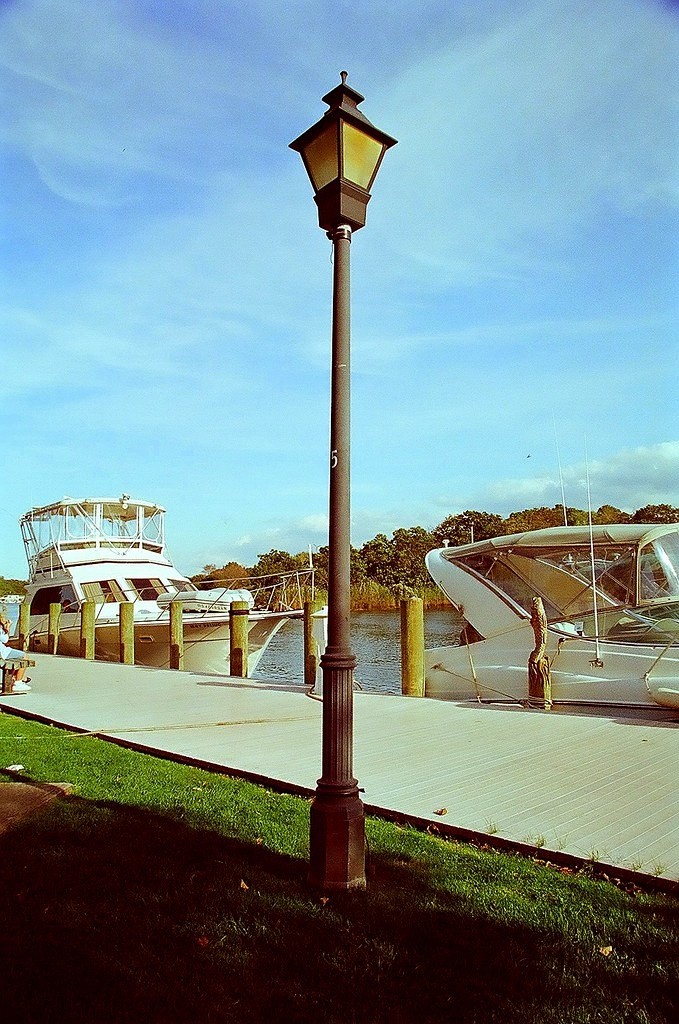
top-left (61, 599), bottom-right (77, 613)
top-left (0, 613), bottom-right (32, 692)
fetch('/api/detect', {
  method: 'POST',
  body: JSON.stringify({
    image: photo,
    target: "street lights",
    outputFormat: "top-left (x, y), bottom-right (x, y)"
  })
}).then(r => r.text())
top-left (288, 69), bottom-right (399, 897)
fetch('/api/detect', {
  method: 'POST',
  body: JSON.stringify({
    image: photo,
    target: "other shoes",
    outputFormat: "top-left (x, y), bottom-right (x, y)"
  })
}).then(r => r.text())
top-left (22, 676), bottom-right (31, 683)
top-left (0, 684), bottom-right (2, 690)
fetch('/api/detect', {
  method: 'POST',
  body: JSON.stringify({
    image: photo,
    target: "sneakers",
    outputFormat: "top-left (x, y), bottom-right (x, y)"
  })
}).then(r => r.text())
top-left (12, 680), bottom-right (31, 691)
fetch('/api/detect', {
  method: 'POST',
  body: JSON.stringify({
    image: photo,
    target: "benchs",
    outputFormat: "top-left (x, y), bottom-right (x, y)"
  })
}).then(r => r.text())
top-left (0, 658), bottom-right (36, 695)
top-left (550, 622), bottom-right (584, 637)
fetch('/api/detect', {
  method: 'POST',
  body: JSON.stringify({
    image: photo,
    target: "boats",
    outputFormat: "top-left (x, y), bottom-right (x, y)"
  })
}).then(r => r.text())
top-left (1, 492), bottom-right (323, 679)
top-left (425, 406), bottom-right (679, 725)
